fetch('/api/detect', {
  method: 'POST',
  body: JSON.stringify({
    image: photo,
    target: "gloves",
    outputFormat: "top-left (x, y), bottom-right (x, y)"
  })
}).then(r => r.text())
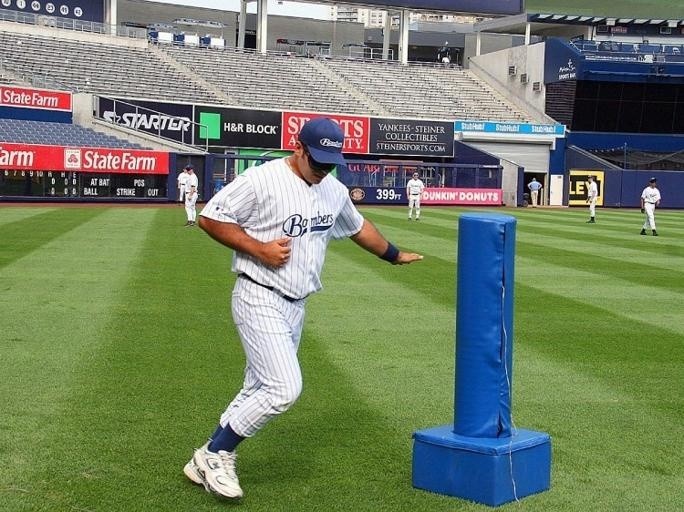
top-left (641, 207), bottom-right (646, 213)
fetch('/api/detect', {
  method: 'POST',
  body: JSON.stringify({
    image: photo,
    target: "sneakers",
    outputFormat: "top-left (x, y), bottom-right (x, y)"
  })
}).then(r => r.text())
top-left (653, 230), bottom-right (658, 236)
top-left (585, 217), bottom-right (596, 223)
top-left (185, 221), bottom-right (195, 227)
top-left (183, 437), bottom-right (244, 502)
top-left (640, 228), bottom-right (647, 235)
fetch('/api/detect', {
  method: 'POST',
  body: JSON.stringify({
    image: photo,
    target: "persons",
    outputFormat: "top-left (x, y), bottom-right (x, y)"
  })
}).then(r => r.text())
top-left (183, 118), bottom-right (424, 501)
top-left (442, 55), bottom-right (450, 68)
top-left (184, 164), bottom-right (198, 227)
top-left (640, 178), bottom-right (661, 236)
top-left (406, 171), bottom-right (424, 220)
top-left (527, 178), bottom-right (542, 208)
top-left (177, 169), bottom-right (190, 203)
top-left (586, 175), bottom-right (598, 223)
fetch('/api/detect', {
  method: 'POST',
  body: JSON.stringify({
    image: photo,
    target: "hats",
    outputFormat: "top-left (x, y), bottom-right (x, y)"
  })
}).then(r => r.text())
top-left (185, 164), bottom-right (195, 169)
top-left (297, 117), bottom-right (348, 169)
top-left (649, 177), bottom-right (657, 182)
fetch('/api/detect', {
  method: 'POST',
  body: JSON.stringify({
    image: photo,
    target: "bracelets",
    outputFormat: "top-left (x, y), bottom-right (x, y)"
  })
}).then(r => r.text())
top-left (380, 242), bottom-right (399, 262)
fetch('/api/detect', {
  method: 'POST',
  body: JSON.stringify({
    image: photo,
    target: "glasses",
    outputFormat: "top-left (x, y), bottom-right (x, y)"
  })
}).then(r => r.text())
top-left (308, 151), bottom-right (336, 170)
top-left (414, 175), bottom-right (418, 176)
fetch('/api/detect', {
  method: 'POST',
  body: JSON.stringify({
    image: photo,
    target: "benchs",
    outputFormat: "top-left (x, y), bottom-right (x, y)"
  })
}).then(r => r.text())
top-left (0, 30), bottom-right (533, 124)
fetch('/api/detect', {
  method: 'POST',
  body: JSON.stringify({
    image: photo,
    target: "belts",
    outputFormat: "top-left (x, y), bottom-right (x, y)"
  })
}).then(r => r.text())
top-left (181, 183), bottom-right (185, 184)
top-left (648, 202), bottom-right (655, 204)
top-left (186, 191), bottom-right (190, 194)
top-left (237, 271), bottom-right (309, 302)
top-left (412, 193), bottom-right (419, 195)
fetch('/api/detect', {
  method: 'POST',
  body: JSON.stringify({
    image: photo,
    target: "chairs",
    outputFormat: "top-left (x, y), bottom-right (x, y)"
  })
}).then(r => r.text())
top-left (0, 114), bottom-right (160, 151)
